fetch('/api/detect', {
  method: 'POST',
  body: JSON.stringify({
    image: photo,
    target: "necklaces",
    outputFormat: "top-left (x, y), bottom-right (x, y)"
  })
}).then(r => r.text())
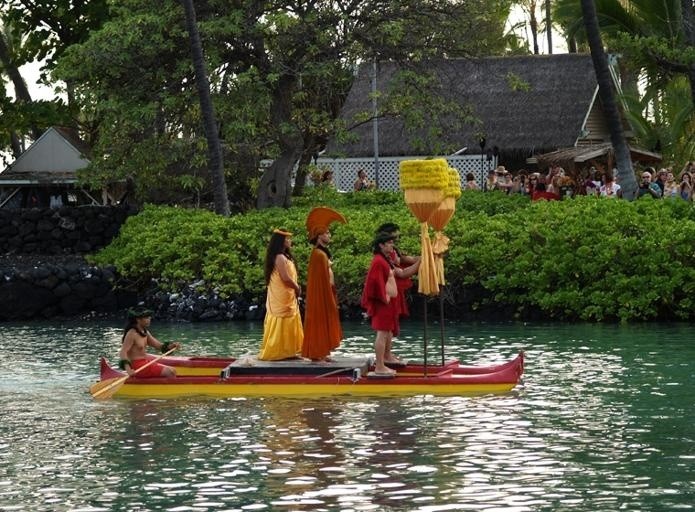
top-left (135, 324), bottom-right (147, 337)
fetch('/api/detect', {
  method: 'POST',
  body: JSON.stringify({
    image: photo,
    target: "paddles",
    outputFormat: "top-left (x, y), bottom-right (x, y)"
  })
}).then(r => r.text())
top-left (91, 348), bottom-right (174, 399)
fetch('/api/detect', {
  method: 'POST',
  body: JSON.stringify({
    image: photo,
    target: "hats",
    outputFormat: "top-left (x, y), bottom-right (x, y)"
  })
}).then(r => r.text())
top-left (495, 165), bottom-right (508, 173)
top-left (322, 170), bottom-right (333, 182)
top-left (373, 232), bottom-right (396, 245)
top-left (307, 207), bottom-right (346, 242)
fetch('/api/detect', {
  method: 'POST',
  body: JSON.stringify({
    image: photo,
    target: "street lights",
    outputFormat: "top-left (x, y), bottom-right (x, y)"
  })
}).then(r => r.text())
top-left (492, 145), bottom-right (500, 170)
top-left (486, 147), bottom-right (492, 170)
top-left (477, 136), bottom-right (487, 190)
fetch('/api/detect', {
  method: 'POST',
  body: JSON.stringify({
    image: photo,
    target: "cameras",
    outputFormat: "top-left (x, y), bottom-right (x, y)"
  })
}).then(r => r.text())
top-left (642, 182), bottom-right (649, 189)
top-left (520, 175), bottom-right (526, 184)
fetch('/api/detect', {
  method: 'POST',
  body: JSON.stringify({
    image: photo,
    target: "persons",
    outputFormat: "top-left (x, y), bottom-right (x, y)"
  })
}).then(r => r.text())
top-left (361, 232), bottom-right (422, 374)
top-left (302, 229), bottom-right (343, 361)
top-left (485, 160), bottom-right (695, 201)
top-left (26, 192), bottom-right (81, 210)
top-left (320, 171), bottom-right (333, 186)
top-left (257, 229), bottom-right (304, 361)
top-left (120, 307), bottom-right (181, 377)
top-left (363, 175), bottom-right (373, 191)
top-left (375, 224), bottom-right (422, 364)
top-left (466, 173), bottom-right (480, 190)
top-left (354, 169), bottom-right (370, 192)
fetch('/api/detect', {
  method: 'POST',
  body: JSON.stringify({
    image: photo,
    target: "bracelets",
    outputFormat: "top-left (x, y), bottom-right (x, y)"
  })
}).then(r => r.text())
top-left (120, 360), bottom-right (131, 369)
top-left (160, 340), bottom-right (172, 355)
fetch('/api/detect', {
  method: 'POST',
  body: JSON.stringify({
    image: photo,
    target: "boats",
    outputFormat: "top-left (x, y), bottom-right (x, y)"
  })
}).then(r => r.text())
top-left (99, 350), bottom-right (528, 399)
